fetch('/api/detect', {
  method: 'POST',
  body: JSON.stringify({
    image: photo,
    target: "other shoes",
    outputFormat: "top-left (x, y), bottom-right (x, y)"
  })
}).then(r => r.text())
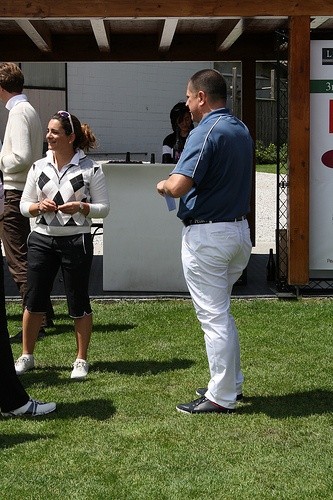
top-left (18, 313), bottom-right (57, 332)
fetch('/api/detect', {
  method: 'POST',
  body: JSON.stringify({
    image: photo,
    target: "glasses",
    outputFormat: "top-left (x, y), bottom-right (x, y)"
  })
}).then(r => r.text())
top-left (58, 111), bottom-right (74, 133)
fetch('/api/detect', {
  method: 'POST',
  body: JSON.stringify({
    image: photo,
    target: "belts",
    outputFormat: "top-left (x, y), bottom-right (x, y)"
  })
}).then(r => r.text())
top-left (182, 217), bottom-right (246, 228)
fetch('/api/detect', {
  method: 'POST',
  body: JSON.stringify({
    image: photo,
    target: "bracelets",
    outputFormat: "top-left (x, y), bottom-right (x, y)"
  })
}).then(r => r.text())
top-left (80, 201), bottom-right (84, 213)
top-left (38, 201), bottom-right (44, 215)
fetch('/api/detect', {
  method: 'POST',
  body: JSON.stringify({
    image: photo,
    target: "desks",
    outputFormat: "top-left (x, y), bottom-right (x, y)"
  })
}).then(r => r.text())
top-left (103, 161), bottom-right (188, 292)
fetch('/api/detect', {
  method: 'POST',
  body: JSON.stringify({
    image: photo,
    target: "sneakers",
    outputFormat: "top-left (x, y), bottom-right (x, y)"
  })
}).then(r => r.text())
top-left (0, 399), bottom-right (56, 417)
top-left (175, 396), bottom-right (236, 415)
top-left (195, 386), bottom-right (243, 402)
top-left (70, 360), bottom-right (89, 378)
top-left (14, 355), bottom-right (35, 375)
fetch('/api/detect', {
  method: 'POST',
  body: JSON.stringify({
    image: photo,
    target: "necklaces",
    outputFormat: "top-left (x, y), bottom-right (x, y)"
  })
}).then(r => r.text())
top-left (58, 159), bottom-right (70, 171)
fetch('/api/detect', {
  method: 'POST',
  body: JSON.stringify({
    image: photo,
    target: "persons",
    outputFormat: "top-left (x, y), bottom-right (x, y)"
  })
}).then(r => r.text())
top-left (162, 101), bottom-right (195, 164)
top-left (0, 62), bottom-right (57, 341)
top-left (14, 111), bottom-right (109, 378)
top-left (0, 170), bottom-right (57, 415)
top-left (156, 69), bottom-right (253, 415)
